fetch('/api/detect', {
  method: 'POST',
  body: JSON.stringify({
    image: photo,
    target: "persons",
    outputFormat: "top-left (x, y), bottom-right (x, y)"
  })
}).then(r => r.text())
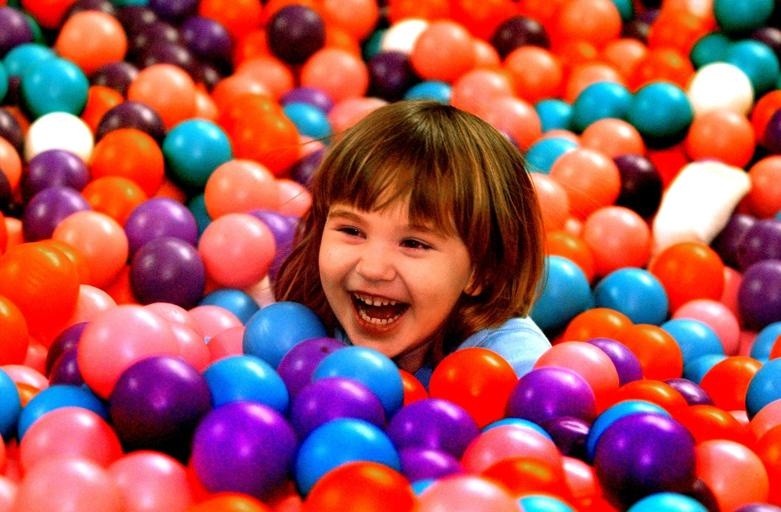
top-left (275, 100), bottom-right (552, 378)
